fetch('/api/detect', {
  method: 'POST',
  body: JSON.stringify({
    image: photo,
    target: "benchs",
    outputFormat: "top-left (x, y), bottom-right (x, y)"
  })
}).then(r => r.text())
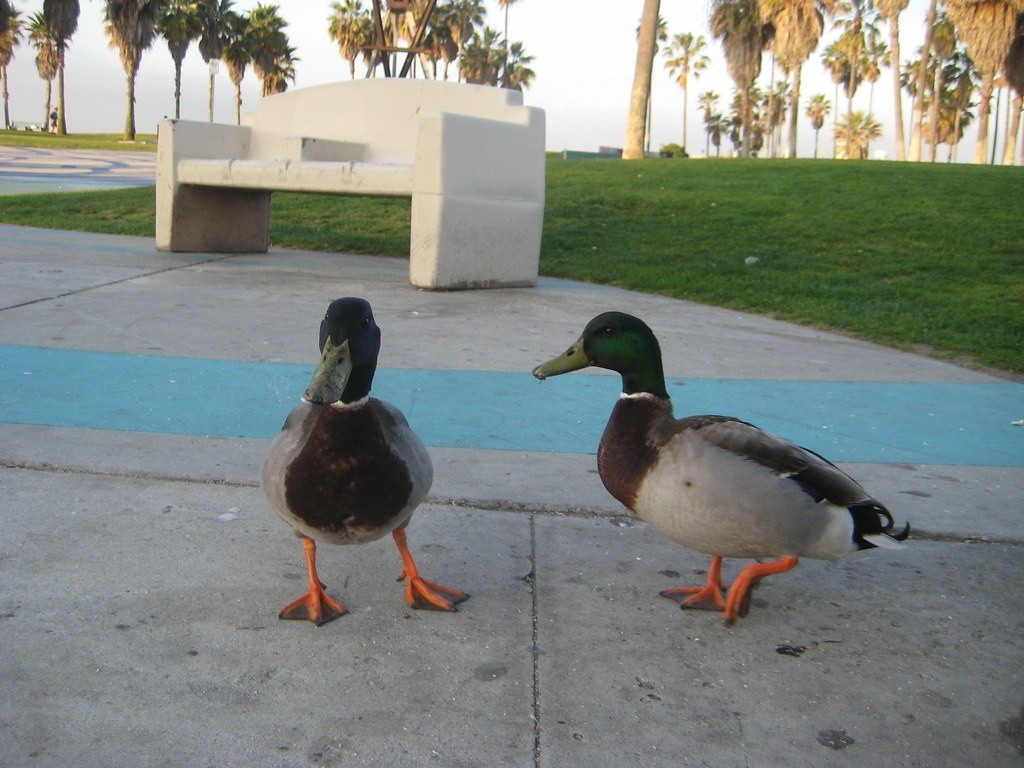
top-left (155, 77), bottom-right (546, 293)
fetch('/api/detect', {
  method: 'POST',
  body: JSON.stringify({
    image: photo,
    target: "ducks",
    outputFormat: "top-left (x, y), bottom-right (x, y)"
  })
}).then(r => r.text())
top-left (265, 296), bottom-right (471, 627)
top-left (532, 309), bottom-right (910, 626)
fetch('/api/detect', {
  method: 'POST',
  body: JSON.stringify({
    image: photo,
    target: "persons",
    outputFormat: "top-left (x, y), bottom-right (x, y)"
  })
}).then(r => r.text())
top-left (50, 107), bottom-right (58, 133)
